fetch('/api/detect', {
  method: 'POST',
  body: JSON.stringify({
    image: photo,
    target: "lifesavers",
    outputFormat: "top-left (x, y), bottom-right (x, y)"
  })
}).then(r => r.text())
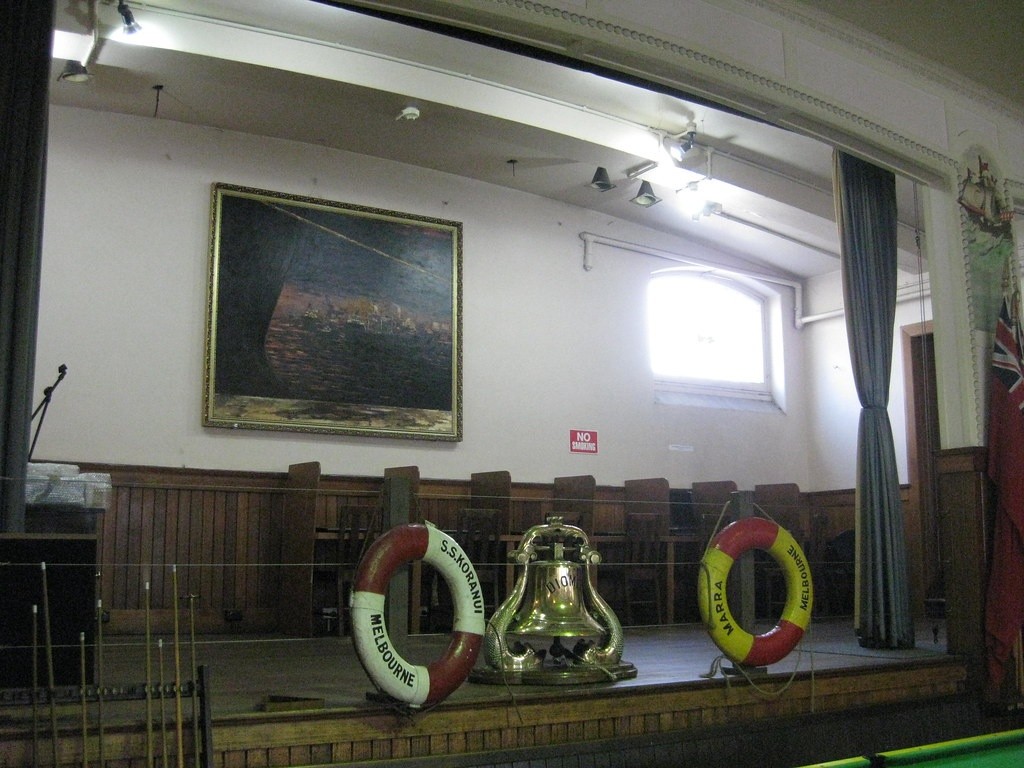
top-left (349, 522), bottom-right (487, 707)
top-left (696, 516), bottom-right (814, 668)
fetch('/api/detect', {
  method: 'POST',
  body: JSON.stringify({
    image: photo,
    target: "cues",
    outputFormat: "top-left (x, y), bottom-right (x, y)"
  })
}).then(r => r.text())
top-left (158, 637), bottom-right (168, 768)
top-left (96, 597), bottom-right (105, 768)
top-left (171, 563), bottom-right (186, 768)
top-left (29, 602), bottom-right (40, 768)
top-left (38, 561), bottom-right (60, 768)
top-left (188, 594), bottom-right (205, 768)
top-left (79, 630), bottom-right (88, 768)
top-left (143, 581), bottom-right (155, 768)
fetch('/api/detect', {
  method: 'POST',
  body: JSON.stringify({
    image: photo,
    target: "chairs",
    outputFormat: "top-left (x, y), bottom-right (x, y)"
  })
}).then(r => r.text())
top-left (424, 504), bottom-right (501, 632)
top-left (313, 505), bottom-right (390, 636)
top-left (763, 536), bottom-right (811, 618)
top-left (545, 511), bottom-right (582, 564)
top-left (599, 511), bottom-right (664, 627)
top-left (681, 513), bottom-right (732, 619)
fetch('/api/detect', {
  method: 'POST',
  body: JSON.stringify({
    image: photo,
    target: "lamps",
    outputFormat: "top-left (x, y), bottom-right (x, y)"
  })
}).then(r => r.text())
top-left (117, 1), bottom-right (141, 33)
top-left (629, 180), bottom-right (664, 208)
top-left (58, 60), bottom-right (96, 84)
top-left (589, 166), bottom-right (617, 194)
top-left (679, 132), bottom-right (696, 153)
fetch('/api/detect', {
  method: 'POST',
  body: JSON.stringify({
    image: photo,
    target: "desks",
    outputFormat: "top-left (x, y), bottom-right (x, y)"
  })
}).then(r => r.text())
top-left (314, 525), bottom-right (795, 635)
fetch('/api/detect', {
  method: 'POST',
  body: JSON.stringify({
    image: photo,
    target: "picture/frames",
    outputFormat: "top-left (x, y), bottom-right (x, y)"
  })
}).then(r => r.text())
top-left (201, 179), bottom-right (463, 444)
